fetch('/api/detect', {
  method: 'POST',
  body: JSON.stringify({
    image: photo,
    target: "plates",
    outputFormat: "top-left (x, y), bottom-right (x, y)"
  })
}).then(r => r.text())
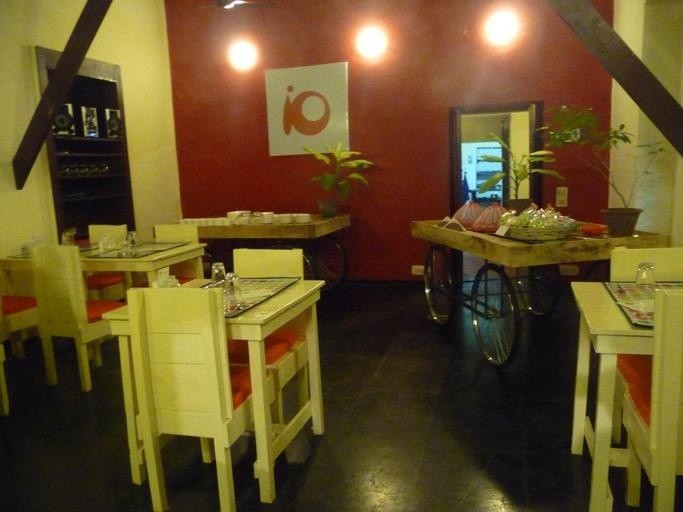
top-left (577, 224), bottom-right (606, 235)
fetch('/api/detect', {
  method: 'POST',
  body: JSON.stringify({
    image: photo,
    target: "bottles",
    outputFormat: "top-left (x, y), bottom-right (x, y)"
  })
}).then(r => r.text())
top-left (149, 272), bottom-right (180, 288)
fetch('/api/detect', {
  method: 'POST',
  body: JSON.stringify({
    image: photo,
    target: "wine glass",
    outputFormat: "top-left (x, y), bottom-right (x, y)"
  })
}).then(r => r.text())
top-left (631, 262), bottom-right (659, 314)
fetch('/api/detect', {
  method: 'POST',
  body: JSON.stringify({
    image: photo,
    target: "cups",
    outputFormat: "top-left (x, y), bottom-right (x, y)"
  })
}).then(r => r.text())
top-left (18, 231), bottom-right (137, 256)
top-left (223, 272), bottom-right (241, 305)
top-left (210, 261), bottom-right (228, 289)
top-left (176, 209), bottom-right (311, 225)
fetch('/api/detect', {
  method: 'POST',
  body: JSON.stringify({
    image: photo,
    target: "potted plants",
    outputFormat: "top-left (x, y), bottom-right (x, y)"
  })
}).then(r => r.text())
top-left (302, 141), bottom-right (374, 218)
top-left (534, 104), bottom-right (671, 236)
top-left (478, 133), bottom-right (565, 217)
